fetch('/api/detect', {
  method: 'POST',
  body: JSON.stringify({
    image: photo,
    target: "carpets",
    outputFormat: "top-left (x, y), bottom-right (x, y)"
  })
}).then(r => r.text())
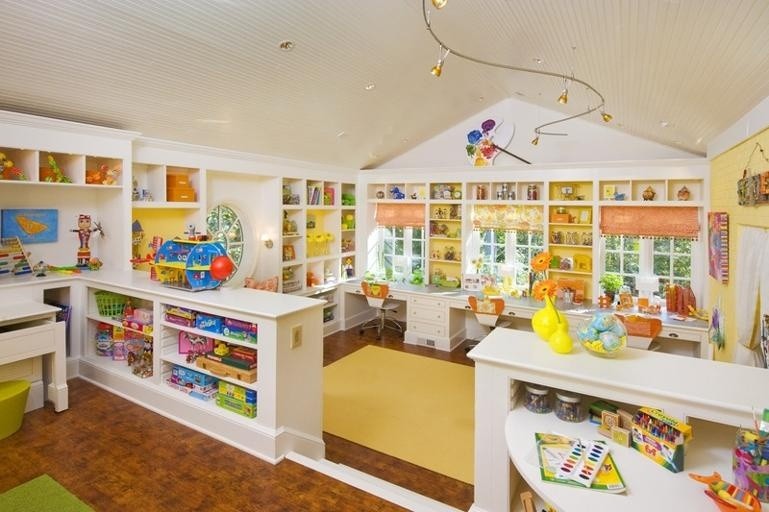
top-left (323, 344), bottom-right (475, 486)
top-left (1, 474), bottom-right (94, 512)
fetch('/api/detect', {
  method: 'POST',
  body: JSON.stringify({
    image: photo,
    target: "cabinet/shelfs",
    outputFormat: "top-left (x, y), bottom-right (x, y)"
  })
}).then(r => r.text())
top-left (467, 328), bottom-right (768, 512)
top-left (0, 110), bottom-right (358, 465)
top-left (360, 159), bottom-right (707, 353)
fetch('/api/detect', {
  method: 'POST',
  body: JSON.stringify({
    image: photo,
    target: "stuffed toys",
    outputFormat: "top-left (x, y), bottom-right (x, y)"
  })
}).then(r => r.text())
top-left (40, 153), bottom-right (73, 184)
top-left (85, 160), bottom-right (119, 186)
top-left (0, 153), bottom-right (30, 181)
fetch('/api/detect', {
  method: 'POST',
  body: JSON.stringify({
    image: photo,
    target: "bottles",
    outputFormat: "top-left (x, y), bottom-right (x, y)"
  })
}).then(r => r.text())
top-left (526, 183), bottom-right (538, 200)
top-left (507, 192), bottom-right (515, 200)
top-left (476, 185), bottom-right (485, 200)
top-left (502, 183), bottom-right (510, 193)
top-left (554, 390), bottom-right (585, 423)
top-left (497, 191), bottom-right (504, 200)
top-left (522, 382), bottom-right (551, 415)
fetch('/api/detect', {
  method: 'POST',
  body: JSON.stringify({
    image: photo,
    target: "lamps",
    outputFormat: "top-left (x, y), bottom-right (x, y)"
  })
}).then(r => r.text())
top-left (422, 0), bottom-right (612, 146)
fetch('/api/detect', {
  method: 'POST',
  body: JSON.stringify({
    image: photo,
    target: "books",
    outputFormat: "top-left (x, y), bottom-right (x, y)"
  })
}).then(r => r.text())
top-left (307, 186), bottom-right (322, 205)
top-left (535, 431), bottom-right (626, 495)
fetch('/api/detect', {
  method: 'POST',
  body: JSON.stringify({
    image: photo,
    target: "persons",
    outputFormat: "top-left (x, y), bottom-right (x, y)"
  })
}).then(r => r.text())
top-left (344, 257), bottom-right (354, 277)
top-left (69, 213), bottom-right (105, 267)
